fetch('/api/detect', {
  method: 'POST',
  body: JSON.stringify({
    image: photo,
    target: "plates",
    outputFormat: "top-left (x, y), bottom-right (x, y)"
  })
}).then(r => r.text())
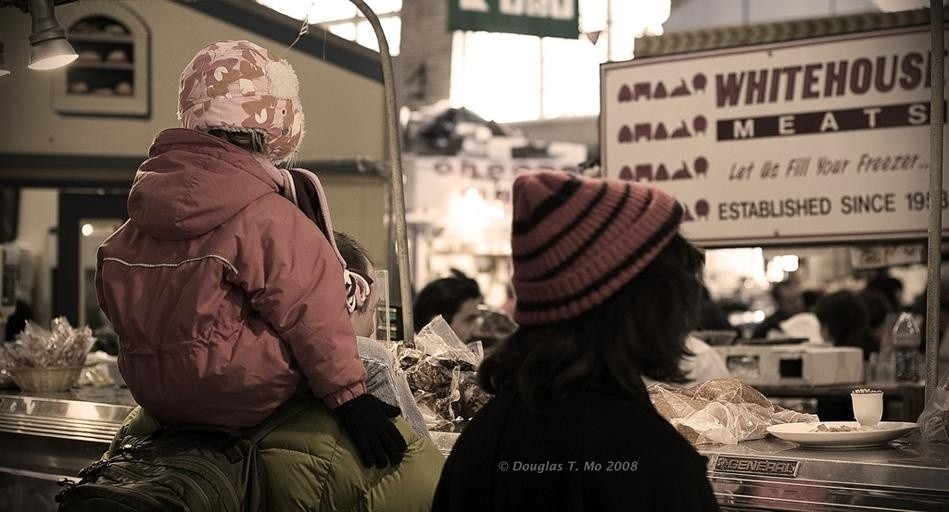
top-left (765, 420), bottom-right (919, 449)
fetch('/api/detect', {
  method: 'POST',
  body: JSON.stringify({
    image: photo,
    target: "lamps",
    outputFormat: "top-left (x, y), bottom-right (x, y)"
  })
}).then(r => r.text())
top-left (27, 0), bottom-right (81, 71)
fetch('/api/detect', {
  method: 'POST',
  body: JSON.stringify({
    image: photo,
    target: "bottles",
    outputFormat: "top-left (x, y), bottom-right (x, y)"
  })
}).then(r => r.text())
top-left (891, 307), bottom-right (922, 385)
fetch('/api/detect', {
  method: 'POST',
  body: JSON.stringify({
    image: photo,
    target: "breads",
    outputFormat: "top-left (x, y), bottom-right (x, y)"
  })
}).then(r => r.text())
top-left (645, 379), bottom-right (817, 449)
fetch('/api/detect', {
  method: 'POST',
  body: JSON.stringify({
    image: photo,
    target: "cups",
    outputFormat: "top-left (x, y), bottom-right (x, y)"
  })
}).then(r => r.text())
top-left (851, 389), bottom-right (885, 430)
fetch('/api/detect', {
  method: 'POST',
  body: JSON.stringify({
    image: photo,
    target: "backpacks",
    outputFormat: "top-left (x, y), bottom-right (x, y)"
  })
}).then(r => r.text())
top-left (55, 380), bottom-right (323, 512)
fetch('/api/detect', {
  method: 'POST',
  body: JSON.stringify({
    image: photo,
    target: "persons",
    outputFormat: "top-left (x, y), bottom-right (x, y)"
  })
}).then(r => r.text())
top-left (412, 276), bottom-right (483, 345)
top-left (92, 38), bottom-right (449, 511)
top-left (747, 276), bottom-right (948, 422)
top-left (428, 169), bottom-right (722, 511)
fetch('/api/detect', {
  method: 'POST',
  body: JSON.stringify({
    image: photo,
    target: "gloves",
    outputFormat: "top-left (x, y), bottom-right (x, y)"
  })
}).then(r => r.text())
top-left (327, 393), bottom-right (409, 470)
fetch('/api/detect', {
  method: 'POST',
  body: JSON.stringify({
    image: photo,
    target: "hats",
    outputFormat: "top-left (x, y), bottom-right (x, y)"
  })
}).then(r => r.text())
top-left (509, 171), bottom-right (684, 329)
top-left (176, 38), bottom-right (306, 165)
